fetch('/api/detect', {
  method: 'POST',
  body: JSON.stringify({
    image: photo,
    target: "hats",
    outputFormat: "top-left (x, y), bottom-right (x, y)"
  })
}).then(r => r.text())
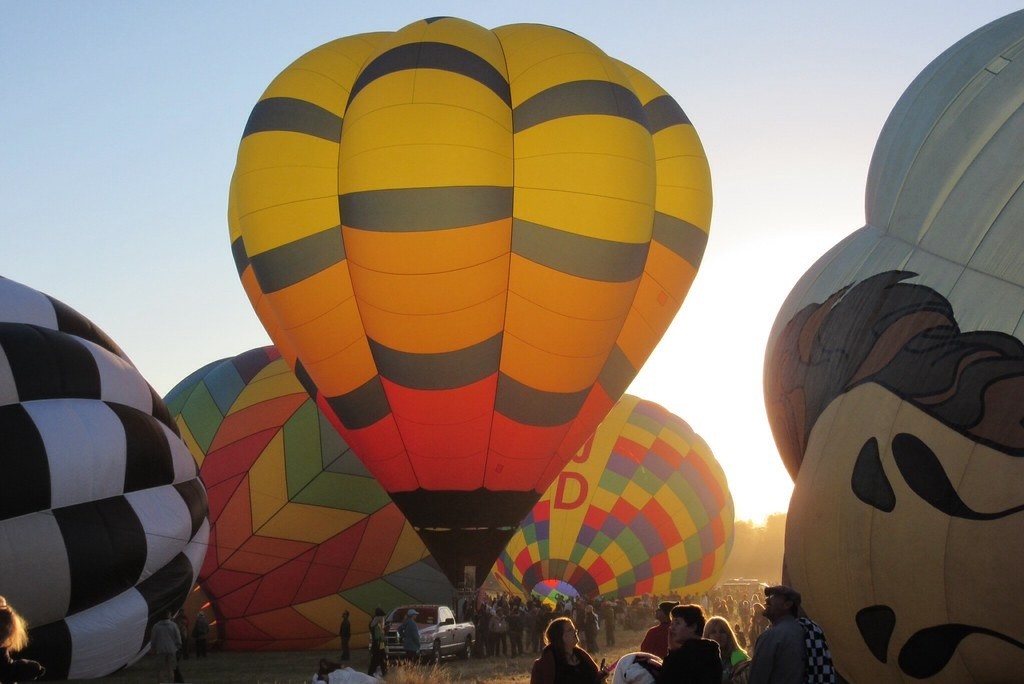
top-left (765, 586), bottom-right (802, 605)
top-left (659, 601), bottom-right (679, 613)
top-left (197, 611), bottom-right (205, 617)
top-left (375, 608), bottom-right (385, 616)
top-left (408, 609), bottom-right (420, 615)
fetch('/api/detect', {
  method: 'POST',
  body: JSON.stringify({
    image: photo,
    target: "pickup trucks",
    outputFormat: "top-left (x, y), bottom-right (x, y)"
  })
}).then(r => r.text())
top-left (368, 604), bottom-right (477, 666)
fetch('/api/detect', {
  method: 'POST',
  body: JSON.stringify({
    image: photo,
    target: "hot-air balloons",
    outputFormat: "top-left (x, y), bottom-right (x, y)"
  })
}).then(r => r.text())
top-left (0, 275), bottom-right (212, 684)
top-left (762, 9), bottom-right (1024, 684)
top-left (227, 14), bottom-right (715, 622)
top-left (487, 393), bottom-right (736, 613)
top-left (161, 344), bottom-right (461, 650)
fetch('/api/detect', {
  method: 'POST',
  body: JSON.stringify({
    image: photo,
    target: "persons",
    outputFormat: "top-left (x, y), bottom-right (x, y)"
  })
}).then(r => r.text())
top-left (529, 617), bottom-right (610, 684)
top-left (461, 592), bottom-right (671, 655)
top-left (0, 597), bottom-right (44, 684)
top-left (319, 608), bottom-right (421, 684)
top-left (612, 584), bottom-right (837, 684)
top-left (150, 606), bottom-right (209, 684)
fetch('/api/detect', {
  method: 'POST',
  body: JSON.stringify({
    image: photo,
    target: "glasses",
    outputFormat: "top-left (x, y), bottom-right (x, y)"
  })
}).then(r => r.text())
top-left (769, 592), bottom-right (784, 599)
